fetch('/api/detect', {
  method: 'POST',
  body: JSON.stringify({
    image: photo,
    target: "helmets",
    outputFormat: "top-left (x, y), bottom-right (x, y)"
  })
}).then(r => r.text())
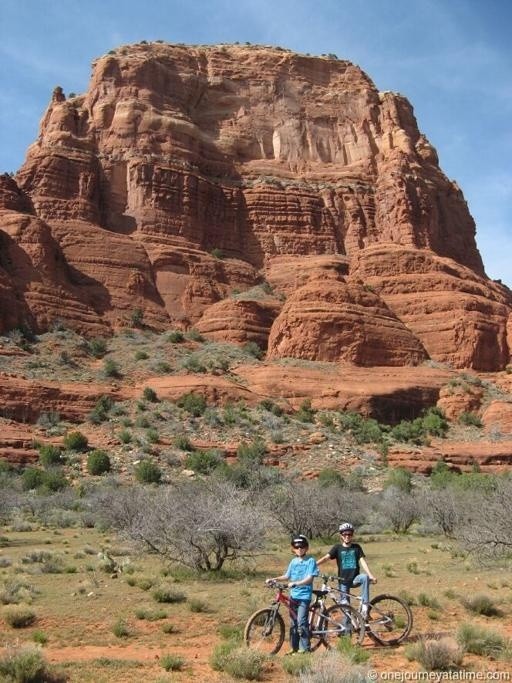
top-left (339, 523), bottom-right (354, 532)
top-left (291, 534), bottom-right (308, 544)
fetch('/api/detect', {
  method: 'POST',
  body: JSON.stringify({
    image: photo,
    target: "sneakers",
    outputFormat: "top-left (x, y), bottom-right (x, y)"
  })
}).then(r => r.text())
top-left (360, 611), bottom-right (371, 620)
top-left (286, 649), bottom-right (305, 656)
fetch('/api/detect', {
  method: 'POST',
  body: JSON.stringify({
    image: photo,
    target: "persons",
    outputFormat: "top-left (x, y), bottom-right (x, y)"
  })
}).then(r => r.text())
top-left (265, 534), bottom-right (320, 657)
top-left (315, 522), bottom-right (378, 638)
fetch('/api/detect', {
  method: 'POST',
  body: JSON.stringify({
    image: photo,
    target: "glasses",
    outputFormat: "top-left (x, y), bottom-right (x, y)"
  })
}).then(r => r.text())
top-left (295, 545), bottom-right (305, 549)
top-left (342, 534), bottom-right (352, 536)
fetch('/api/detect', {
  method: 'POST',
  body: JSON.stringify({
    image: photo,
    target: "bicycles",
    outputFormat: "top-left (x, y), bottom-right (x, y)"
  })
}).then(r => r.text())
top-left (307, 574), bottom-right (412, 646)
top-left (244, 581), bottom-right (365, 657)
